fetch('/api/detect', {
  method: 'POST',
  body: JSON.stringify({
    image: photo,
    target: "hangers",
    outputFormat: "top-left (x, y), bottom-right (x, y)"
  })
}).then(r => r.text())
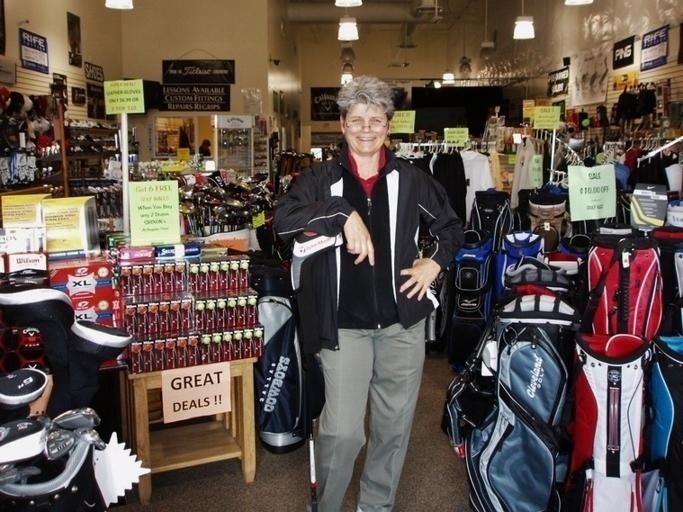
top-left (519, 82), bottom-right (683, 187)
top-left (394, 139), bottom-right (498, 160)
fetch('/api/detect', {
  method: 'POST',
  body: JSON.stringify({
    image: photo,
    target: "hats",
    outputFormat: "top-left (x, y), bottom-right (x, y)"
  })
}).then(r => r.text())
top-left (0, 85), bottom-right (50, 138)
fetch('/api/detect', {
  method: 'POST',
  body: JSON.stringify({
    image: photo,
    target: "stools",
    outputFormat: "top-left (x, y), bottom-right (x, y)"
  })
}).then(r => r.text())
top-left (120, 357), bottom-right (260, 505)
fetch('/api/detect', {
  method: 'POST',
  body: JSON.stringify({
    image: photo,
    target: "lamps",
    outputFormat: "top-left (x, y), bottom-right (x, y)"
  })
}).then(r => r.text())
top-left (442, 0), bottom-right (594, 85)
top-left (333, 0), bottom-right (363, 88)
top-left (105, 0), bottom-right (133, 11)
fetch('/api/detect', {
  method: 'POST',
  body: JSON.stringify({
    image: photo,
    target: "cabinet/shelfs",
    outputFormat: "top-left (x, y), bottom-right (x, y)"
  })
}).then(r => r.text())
top-left (0, 85), bottom-right (69, 197)
top-left (63, 119), bottom-right (123, 218)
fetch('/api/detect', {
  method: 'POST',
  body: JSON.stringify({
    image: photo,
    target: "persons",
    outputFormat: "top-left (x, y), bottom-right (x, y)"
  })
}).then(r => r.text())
top-left (197, 139), bottom-right (211, 157)
top-left (270, 74), bottom-right (465, 512)
